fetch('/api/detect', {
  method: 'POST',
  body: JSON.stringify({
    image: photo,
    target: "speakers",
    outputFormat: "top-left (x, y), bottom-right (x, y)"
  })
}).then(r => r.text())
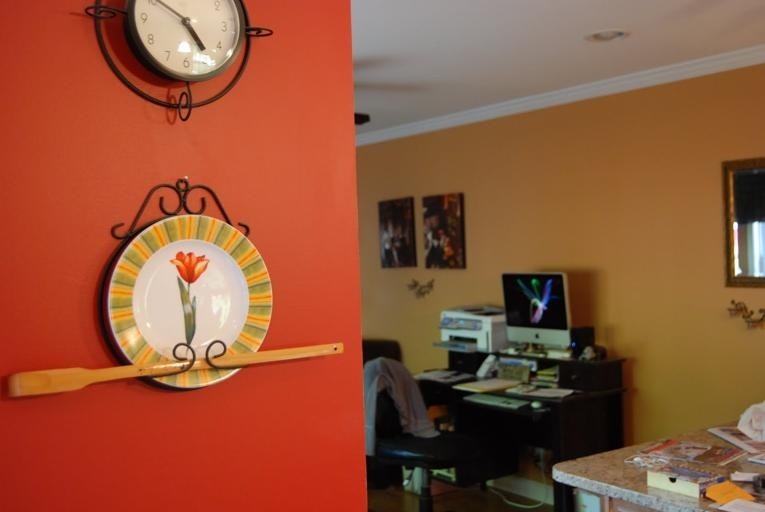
top-left (570, 326), bottom-right (594, 358)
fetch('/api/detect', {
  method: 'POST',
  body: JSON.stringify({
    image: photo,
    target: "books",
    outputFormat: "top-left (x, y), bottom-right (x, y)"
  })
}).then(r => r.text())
top-left (641, 436), bottom-right (746, 466)
top-left (646, 467), bottom-right (725, 501)
top-left (707, 426), bottom-right (764, 453)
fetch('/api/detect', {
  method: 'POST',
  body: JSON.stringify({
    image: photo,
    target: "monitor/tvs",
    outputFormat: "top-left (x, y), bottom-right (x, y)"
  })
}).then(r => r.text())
top-left (502, 272), bottom-right (571, 356)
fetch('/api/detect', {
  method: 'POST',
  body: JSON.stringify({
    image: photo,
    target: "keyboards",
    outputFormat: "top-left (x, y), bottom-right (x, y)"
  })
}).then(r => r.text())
top-left (463, 393), bottom-right (531, 410)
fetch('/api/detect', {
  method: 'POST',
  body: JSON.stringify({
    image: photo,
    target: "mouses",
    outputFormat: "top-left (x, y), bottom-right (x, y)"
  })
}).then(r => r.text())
top-left (532, 400), bottom-right (542, 409)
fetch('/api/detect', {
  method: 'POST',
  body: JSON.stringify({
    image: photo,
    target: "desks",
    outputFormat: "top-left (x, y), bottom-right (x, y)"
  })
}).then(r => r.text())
top-left (410, 349), bottom-right (629, 512)
top-left (553, 438), bottom-right (727, 512)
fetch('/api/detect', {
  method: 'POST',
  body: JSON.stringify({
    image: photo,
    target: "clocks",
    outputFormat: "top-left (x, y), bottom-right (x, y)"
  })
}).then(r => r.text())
top-left (81, 0), bottom-right (276, 124)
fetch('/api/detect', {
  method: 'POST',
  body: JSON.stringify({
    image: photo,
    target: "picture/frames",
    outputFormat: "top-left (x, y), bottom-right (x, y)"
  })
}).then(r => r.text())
top-left (420, 192), bottom-right (465, 271)
top-left (376, 194), bottom-right (418, 270)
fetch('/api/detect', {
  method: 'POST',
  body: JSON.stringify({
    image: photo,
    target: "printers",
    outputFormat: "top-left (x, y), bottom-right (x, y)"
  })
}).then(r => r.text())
top-left (433, 304), bottom-right (508, 354)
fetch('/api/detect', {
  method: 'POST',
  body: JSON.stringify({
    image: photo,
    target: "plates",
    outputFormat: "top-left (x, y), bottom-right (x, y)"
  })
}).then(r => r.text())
top-left (104, 212), bottom-right (275, 390)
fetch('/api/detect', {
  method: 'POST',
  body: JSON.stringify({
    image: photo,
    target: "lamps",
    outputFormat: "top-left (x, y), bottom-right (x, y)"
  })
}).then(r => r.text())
top-left (583, 29), bottom-right (631, 45)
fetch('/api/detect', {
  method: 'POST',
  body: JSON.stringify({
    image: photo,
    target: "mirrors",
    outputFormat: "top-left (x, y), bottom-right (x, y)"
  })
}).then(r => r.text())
top-left (720, 155), bottom-right (765, 289)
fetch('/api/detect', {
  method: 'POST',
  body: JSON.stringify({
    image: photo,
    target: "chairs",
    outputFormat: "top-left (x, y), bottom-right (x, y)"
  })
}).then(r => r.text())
top-left (363, 357), bottom-right (477, 512)
